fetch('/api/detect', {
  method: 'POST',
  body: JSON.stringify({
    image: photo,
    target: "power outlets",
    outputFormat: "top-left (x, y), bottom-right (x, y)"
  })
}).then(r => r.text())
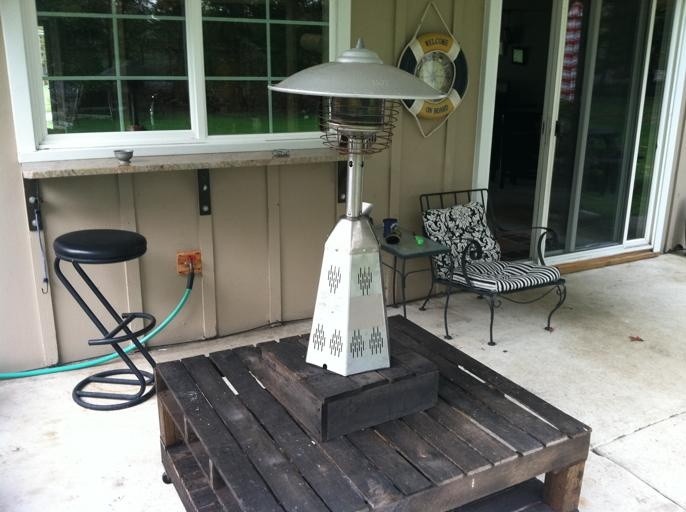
top-left (512, 48), bottom-right (524, 64)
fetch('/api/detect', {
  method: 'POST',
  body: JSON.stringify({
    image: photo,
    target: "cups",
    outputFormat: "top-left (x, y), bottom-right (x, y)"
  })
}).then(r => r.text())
top-left (382, 218), bottom-right (398, 238)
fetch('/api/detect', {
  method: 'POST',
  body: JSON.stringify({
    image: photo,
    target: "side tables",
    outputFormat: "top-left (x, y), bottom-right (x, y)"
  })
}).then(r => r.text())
top-left (372, 224), bottom-right (456, 340)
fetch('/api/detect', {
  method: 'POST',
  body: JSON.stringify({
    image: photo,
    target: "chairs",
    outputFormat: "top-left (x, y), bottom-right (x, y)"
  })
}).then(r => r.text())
top-left (419, 188), bottom-right (567, 347)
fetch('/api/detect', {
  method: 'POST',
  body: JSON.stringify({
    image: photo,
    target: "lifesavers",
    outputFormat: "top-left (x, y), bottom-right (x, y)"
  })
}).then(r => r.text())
top-left (397, 33), bottom-right (468, 120)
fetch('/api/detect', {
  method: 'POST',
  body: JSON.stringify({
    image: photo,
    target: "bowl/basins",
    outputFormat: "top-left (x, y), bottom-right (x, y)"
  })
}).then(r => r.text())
top-left (113, 148), bottom-right (134, 164)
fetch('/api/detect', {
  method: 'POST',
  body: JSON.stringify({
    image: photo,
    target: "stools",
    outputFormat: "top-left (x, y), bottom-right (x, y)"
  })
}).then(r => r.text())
top-left (51, 229), bottom-right (156, 410)
top-left (497, 112), bottom-right (540, 188)
